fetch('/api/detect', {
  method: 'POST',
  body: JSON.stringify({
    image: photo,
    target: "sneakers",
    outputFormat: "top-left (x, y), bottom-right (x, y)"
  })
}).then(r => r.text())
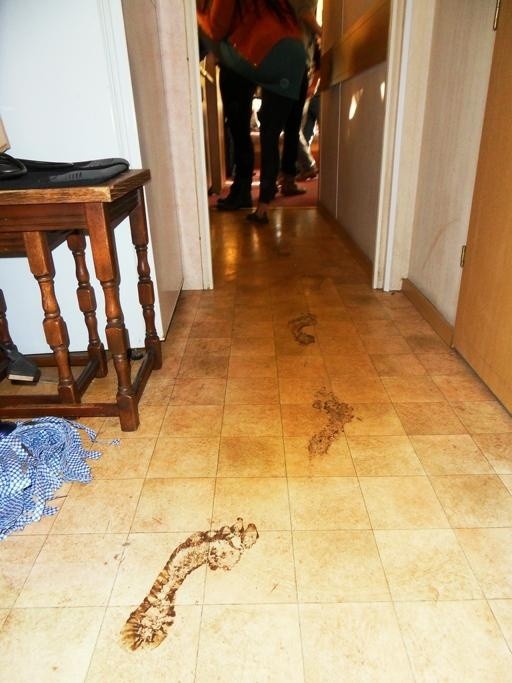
top-left (247, 211), bottom-right (268, 224)
top-left (295, 167), bottom-right (316, 181)
top-left (281, 184), bottom-right (306, 194)
top-left (216, 192), bottom-right (253, 210)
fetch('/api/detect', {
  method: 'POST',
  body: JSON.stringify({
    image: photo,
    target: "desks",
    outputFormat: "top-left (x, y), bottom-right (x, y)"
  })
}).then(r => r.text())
top-left (3, 165), bottom-right (167, 432)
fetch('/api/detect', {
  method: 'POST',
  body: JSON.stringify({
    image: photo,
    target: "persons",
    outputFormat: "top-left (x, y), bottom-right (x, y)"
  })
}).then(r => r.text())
top-left (197, 0), bottom-right (323, 224)
